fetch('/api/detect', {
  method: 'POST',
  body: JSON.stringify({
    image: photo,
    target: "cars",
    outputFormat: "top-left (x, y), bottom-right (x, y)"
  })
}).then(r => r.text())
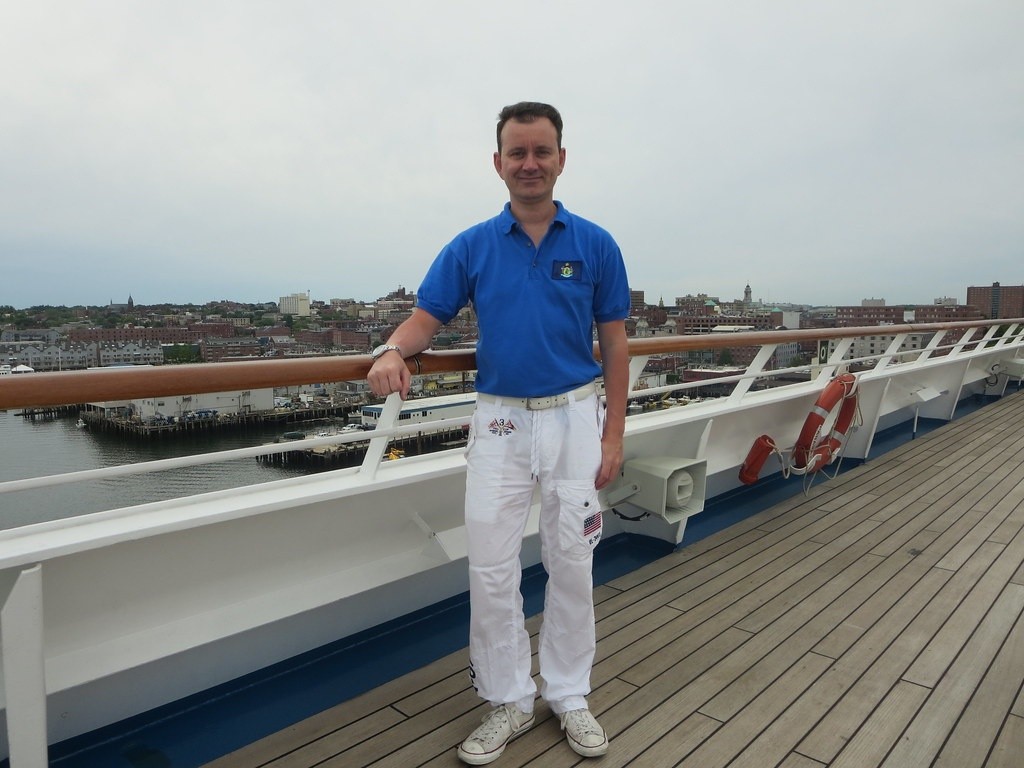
top-left (318, 399), bottom-right (331, 404)
top-left (342, 423), bottom-right (355, 430)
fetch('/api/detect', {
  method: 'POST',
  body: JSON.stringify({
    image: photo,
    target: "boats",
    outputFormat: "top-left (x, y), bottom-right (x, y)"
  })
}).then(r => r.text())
top-left (75, 418), bottom-right (87, 428)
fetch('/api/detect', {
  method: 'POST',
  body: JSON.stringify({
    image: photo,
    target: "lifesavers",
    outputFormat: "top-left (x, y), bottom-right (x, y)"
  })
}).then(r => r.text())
top-left (794, 373), bottom-right (859, 475)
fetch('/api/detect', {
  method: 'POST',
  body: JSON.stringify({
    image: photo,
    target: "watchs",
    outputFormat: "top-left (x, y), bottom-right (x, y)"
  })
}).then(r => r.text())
top-left (371, 345), bottom-right (401, 362)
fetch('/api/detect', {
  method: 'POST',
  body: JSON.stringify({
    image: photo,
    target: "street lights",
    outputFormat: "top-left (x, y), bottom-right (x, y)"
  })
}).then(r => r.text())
top-left (59, 346), bottom-right (62, 371)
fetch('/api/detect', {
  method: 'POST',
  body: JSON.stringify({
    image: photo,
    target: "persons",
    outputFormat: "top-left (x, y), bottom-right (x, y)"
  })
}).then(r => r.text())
top-left (367, 102), bottom-right (631, 764)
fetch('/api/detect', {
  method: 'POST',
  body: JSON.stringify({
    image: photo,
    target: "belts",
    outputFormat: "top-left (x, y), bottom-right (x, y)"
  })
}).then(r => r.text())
top-left (477, 381), bottom-right (596, 410)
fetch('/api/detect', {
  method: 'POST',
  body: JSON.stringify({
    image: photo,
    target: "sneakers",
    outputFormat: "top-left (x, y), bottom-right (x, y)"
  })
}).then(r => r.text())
top-left (553, 703), bottom-right (609, 757)
top-left (457, 702), bottom-right (536, 765)
